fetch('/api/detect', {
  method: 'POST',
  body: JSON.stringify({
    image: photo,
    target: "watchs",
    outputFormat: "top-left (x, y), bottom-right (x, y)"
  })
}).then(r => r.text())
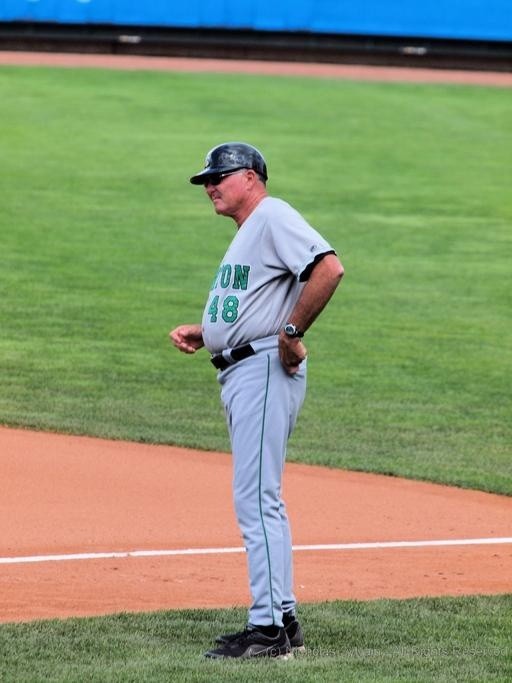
top-left (284, 322), bottom-right (305, 339)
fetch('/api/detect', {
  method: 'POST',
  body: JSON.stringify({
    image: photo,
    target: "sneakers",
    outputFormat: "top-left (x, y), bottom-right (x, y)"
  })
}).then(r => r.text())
top-left (205, 625), bottom-right (293, 661)
top-left (216, 619), bottom-right (306, 651)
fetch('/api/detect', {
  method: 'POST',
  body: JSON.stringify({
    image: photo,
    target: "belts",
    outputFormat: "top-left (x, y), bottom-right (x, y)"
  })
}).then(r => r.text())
top-left (210, 345), bottom-right (254, 371)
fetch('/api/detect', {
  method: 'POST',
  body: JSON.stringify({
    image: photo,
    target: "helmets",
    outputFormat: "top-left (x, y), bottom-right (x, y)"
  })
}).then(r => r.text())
top-left (188, 140), bottom-right (269, 186)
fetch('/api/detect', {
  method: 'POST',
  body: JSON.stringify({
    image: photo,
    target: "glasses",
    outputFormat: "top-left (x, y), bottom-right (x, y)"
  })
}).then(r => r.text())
top-left (202, 169), bottom-right (245, 187)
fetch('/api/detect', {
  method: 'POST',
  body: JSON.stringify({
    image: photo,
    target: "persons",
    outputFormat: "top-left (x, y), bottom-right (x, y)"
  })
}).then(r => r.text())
top-left (166, 139), bottom-right (345, 666)
